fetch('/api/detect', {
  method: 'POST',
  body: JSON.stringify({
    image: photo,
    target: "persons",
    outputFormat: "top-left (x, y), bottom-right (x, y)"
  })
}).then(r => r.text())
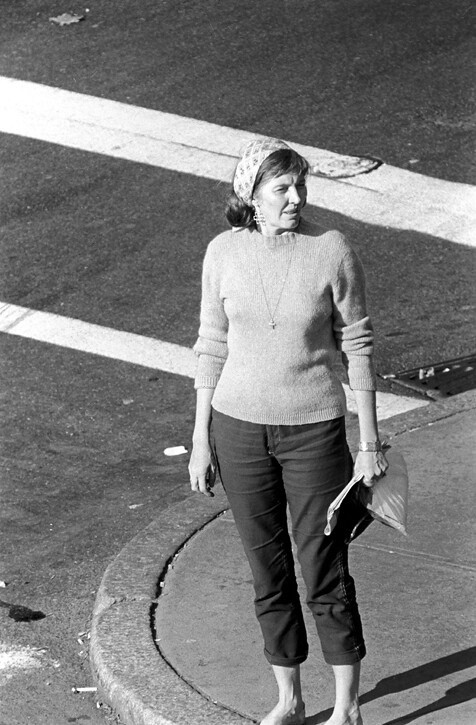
top-left (187, 140), bottom-right (388, 725)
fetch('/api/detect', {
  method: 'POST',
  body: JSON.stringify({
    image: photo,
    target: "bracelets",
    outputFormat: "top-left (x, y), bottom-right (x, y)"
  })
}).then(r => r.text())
top-left (358, 439), bottom-right (382, 451)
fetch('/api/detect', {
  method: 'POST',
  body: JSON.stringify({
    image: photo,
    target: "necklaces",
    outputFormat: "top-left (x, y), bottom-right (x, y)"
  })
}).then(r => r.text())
top-left (254, 229), bottom-right (299, 329)
